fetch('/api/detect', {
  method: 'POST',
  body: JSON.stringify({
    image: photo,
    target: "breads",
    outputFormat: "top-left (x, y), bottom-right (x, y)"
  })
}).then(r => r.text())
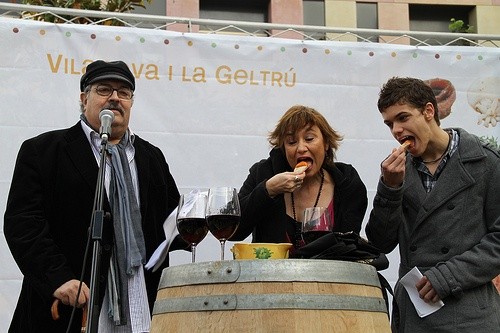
top-left (294, 165), bottom-right (309, 173)
top-left (399, 140), bottom-right (411, 151)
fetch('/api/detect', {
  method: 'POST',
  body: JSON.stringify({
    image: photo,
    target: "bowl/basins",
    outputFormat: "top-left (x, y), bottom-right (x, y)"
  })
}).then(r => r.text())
top-left (233, 243), bottom-right (293, 260)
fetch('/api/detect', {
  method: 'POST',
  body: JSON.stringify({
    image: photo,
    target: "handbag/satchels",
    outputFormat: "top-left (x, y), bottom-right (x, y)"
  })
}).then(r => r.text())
top-left (292, 229), bottom-right (389, 271)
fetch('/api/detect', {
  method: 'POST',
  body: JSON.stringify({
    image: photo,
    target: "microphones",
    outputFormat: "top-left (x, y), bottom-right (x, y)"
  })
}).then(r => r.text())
top-left (98, 109), bottom-right (115, 145)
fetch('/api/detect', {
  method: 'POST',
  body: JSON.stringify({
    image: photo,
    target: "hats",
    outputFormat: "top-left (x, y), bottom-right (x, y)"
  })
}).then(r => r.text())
top-left (80, 59), bottom-right (135, 93)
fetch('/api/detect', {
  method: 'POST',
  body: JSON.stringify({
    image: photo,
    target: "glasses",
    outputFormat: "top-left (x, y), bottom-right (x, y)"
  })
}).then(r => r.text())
top-left (91, 84), bottom-right (134, 100)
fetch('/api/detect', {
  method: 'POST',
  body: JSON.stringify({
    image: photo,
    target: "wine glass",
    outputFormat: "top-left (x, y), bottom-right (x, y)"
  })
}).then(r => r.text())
top-left (205, 187), bottom-right (241, 260)
top-left (176, 193), bottom-right (209, 263)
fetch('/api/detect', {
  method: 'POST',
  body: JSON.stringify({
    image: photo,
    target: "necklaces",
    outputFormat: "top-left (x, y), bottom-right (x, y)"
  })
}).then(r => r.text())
top-left (421, 131), bottom-right (451, 164)
top-left (290, 168), bottom-right (328, 250)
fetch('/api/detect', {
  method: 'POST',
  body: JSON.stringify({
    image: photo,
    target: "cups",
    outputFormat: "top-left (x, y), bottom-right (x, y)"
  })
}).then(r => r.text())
top-left (301, 207), bottom-right (331, 246)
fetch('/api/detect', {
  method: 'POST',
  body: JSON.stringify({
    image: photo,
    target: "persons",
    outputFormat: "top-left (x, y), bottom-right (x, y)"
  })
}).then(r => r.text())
top-left (4, 57), bottom-right (180, 333)
top-left (215, 105), bottom-right (368, 258)
top-left (366, 76), bottom-right (499, 332)
